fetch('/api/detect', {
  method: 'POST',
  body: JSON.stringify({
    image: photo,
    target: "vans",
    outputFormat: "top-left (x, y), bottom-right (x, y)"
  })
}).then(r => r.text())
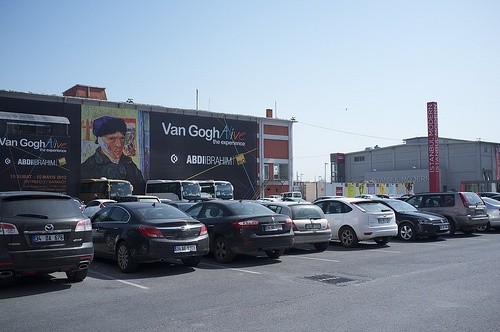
top-left (281, 191), bottom-right (303, 200)
top-left (405, 190), bottom-right (490, 236)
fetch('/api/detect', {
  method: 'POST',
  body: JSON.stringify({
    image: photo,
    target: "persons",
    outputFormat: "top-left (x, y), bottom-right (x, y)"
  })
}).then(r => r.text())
top-left (80, 115), bottom-right (145, 195)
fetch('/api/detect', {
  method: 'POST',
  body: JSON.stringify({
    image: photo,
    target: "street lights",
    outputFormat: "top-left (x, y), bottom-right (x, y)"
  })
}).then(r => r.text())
top-left (325, 163), bottom-right (328, 196)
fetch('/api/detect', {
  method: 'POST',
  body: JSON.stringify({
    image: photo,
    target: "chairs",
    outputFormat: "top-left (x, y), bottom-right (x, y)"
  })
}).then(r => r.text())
top-left (210, 208), bottom-right (220, 216)
top-left (329, 205), bottom-right (337, 214)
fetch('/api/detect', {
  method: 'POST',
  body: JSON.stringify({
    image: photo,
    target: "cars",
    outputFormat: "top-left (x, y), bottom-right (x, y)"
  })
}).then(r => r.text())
top-left (312, 194), bottom-right (450, 248)
top-left (256, 197), bottom-right (332, 252)
top-left (82, 198), bottom-right (295, 274)
top-left (475, 192), bottom-right (500, 232)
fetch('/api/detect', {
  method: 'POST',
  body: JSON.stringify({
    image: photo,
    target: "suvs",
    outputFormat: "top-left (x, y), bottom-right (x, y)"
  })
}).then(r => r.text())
top-left (0, 191), bottom-right (95, 288)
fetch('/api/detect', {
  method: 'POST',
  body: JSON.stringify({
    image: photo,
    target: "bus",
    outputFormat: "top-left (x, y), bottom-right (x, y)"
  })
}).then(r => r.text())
top-left (79, 177), bottom-right (133, 206)
top-left (144, 178), bottom-right (234, 203)
